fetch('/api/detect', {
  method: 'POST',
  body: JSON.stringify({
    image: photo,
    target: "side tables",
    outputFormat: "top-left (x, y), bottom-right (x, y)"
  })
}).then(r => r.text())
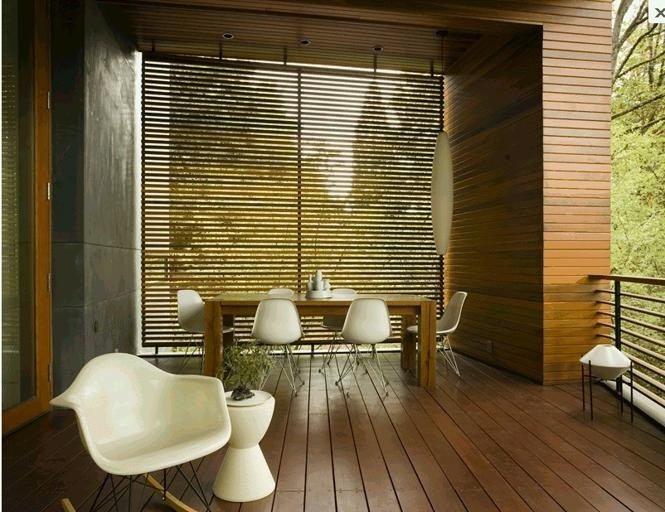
top-left (211, 390), bottom-right (275, 502)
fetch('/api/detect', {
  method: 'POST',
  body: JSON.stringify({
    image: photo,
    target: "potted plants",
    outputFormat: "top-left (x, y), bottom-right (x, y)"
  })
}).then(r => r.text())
top-left (212, 339), bottom-right (276, 400)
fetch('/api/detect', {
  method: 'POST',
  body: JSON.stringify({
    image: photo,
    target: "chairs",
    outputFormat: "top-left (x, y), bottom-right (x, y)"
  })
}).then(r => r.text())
top-left (268, 288), bottom-right (311, 373)
top-left (177, 290), bottom-right (236, 376)
top-left (318, 289), bottom-right (368, 374)
top-left (49, 353), bottom-right (232, 512)
top-left (408, 292), bottom-right (468, 377)
top-left (251, 297), bottom-right (304, 397)
top-left (335, 298), bottom-right (392, 397)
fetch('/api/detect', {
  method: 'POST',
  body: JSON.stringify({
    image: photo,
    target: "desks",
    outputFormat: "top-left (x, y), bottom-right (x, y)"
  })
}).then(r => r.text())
top-left (205, 294), bottom-right (437, 388)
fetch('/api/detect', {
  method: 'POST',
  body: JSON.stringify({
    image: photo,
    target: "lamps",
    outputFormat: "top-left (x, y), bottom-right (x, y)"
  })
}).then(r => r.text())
top-left (431, 31), bottom-right (454, 255)
